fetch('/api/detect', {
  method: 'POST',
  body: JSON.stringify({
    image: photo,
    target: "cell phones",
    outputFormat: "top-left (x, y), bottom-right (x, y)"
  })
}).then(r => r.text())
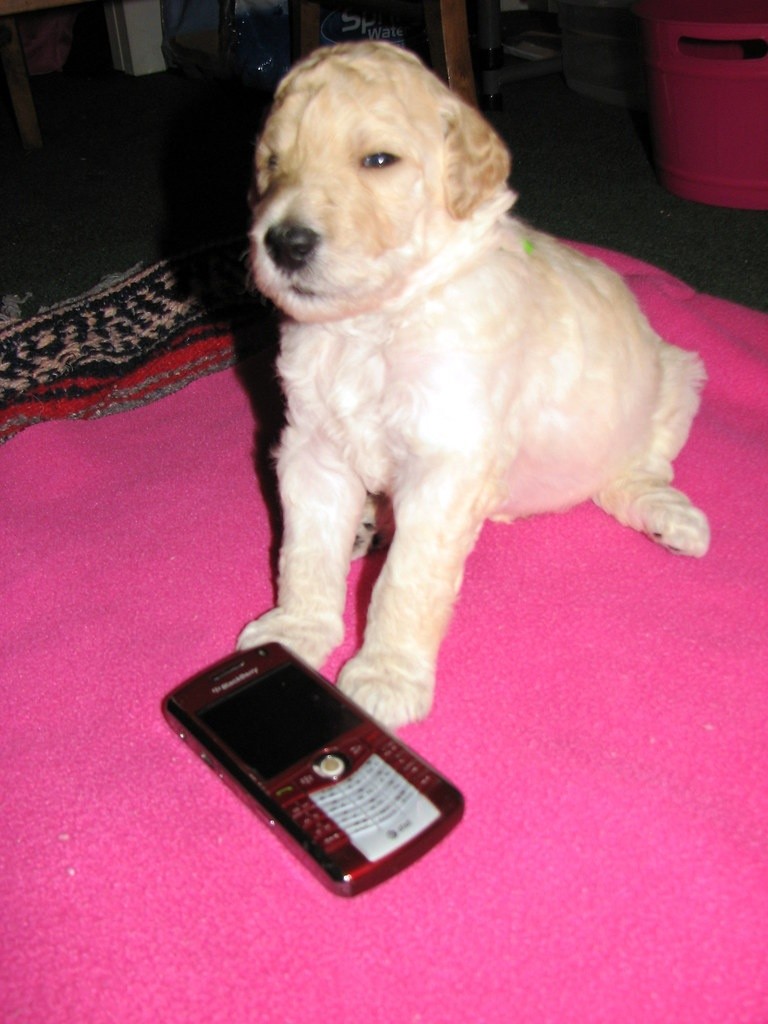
top-left (158, 641), bottom-right (465, 899)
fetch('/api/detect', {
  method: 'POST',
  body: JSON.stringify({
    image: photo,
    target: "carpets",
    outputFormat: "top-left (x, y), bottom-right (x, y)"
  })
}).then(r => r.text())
top-left (0, 233), bottom-right (768, 1024)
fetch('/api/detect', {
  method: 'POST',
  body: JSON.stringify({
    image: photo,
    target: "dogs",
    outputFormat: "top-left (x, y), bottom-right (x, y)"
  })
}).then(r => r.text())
top-left (229, 37), bottom-right (713, 738)
